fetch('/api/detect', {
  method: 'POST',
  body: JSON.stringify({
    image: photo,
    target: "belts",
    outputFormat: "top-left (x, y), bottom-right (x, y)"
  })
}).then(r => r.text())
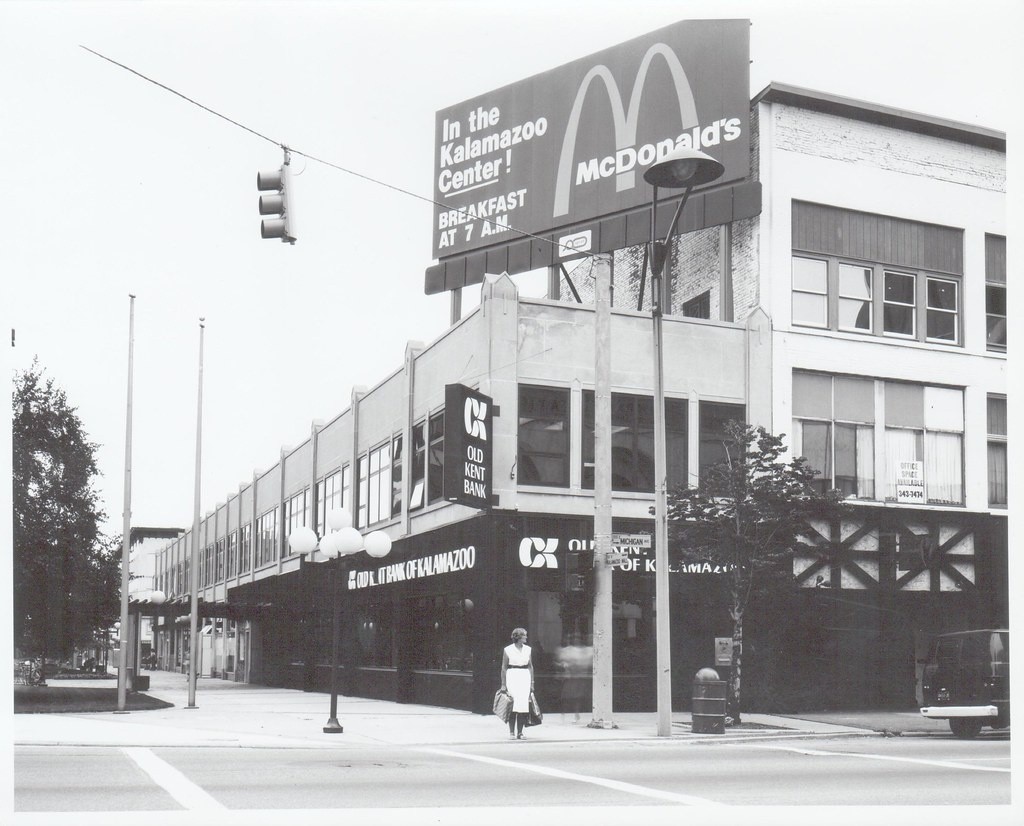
top-left (507, 664), bottom-right (529, 669)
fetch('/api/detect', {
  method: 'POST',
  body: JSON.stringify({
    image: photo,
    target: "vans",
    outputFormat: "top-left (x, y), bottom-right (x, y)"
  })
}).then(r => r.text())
top-left (920, 628), bottom-right (1010, 739)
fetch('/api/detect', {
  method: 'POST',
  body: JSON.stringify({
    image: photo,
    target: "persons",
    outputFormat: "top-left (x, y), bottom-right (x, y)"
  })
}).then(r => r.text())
top-left (501, 628), bottom-right (535, 740)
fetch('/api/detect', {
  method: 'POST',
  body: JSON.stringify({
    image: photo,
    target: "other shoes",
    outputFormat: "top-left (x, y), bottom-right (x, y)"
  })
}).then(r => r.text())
top-left (509, 733), bottom-right (515, 740)
top-left (517, 735), bottom-right (526, 740)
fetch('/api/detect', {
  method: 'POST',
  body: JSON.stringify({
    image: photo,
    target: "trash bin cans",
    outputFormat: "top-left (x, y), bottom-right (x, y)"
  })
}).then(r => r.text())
top-left (691, 679), bottom-right (728, 734)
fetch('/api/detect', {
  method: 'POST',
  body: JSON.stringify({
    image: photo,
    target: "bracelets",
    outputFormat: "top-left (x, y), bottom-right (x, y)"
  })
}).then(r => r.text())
top-left (531, 681), bottom-right (535, 683)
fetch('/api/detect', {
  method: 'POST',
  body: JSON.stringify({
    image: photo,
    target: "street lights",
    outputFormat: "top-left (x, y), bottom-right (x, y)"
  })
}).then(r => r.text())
top-left (643, 145), bottom-right (727, 738)
top-left (289, 507), bottom-right (392, 734)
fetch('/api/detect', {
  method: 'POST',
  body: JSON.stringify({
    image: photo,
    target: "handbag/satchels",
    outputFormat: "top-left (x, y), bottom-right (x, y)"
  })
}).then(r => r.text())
top-left (493, 689), bottom-right (514, 723)
top-left (525, 692), bottom-right (542, 727)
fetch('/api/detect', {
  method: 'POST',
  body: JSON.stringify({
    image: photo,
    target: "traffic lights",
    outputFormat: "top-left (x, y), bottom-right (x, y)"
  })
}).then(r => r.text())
top-left (257, 163), bottom-right (293, 242)
